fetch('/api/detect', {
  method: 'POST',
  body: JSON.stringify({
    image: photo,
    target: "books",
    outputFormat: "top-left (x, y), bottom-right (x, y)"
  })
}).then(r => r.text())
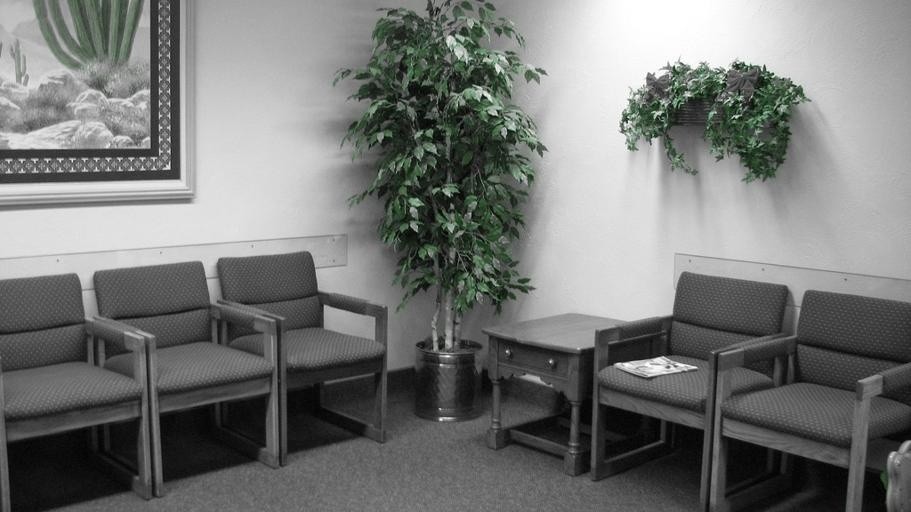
top-left (615, 355), bottom-right (698, 379)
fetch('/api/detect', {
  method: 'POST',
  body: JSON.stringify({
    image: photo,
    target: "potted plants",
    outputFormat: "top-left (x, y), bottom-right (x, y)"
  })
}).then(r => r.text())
top-left (332, 0), bottom-right (548, 424)
top-left (619, 58), bottom-right (811, 184)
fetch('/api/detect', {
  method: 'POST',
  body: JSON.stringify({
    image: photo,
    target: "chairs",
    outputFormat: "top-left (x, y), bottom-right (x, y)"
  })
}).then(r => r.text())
top-left (1, 273), bottom-right (152, 510)
top-left (93, 262), bottom-right (281, 498)
top-left (589, 272), bottom-right (788, 511)
top-left (218, 251), bottom-right (388, 466)
top-left (709, 289), bottom-right (911, 512)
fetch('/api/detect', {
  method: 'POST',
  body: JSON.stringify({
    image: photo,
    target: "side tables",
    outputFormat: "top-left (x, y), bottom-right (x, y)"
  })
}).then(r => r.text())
top-left (481, 312), bottom-right (630, 477)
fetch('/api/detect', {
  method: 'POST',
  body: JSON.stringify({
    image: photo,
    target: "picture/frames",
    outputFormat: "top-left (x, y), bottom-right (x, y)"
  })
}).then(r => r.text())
top-left (0, 0), bottom-right (196, 205)
